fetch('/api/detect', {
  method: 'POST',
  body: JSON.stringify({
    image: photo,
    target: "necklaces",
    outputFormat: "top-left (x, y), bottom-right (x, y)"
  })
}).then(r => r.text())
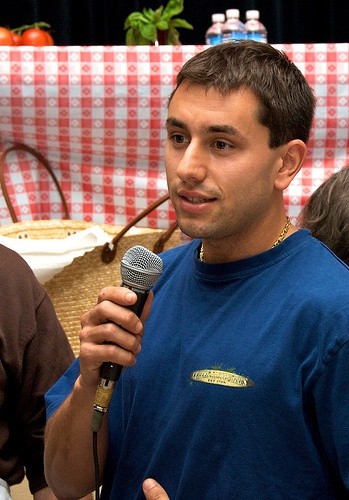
top-left (200, 215), bottom-right (291, 261)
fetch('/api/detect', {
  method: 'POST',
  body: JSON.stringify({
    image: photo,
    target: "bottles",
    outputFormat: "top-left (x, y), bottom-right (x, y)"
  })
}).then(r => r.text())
top-left (206, 13), bottom-right (225, 45)
top-left (220, 9), bottom-right (248, 44)
top-left (243, 10), bottom-right (268, 44)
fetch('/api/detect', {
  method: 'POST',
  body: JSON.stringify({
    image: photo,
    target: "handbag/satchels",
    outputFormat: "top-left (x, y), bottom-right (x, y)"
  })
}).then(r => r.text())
top-left (0, 145), bottom-right (178, 409)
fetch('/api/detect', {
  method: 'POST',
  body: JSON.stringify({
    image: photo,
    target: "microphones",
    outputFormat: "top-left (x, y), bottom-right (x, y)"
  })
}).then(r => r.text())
top-left (91, 246), bottom-right (164, 432)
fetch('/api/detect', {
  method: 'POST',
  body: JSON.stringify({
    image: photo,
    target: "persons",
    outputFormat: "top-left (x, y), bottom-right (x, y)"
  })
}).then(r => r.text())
top-left (0, 244), bottom-right (95, 500)
top-left (296, 164), bottom-right (349, 264)
top-left (42, 39), bottom-right (349, 500)
